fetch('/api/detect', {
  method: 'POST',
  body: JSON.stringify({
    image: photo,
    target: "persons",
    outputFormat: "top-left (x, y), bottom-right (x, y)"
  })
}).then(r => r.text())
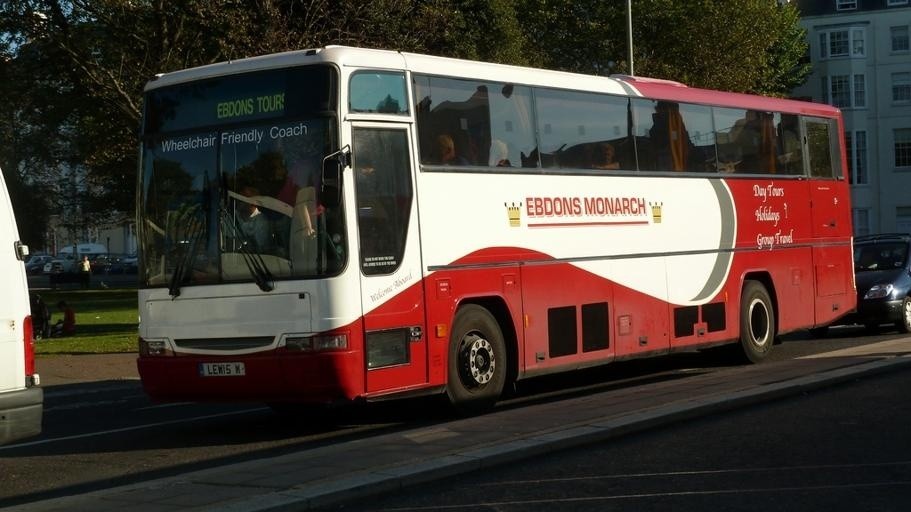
top-left (590, 141), bottom-right (622, 171)
top-left (433, 132), bottom-right (456, 165)
top-left (487, 139), bottom-right (512, 168)
top-left (271, 131), bottom-right (328, 257)
top-left (78, 255), bottom-right (91, 290)
top-left (30, 292), bottom-right (52, 339)
top-left (53, 300), bottom-right (76, 338)
top-left (231, 185), bottom-right (277, 257)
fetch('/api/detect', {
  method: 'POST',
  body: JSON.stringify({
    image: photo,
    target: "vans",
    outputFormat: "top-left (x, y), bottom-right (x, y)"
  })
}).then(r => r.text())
top-left (0, 170), bottom-right (44, 445)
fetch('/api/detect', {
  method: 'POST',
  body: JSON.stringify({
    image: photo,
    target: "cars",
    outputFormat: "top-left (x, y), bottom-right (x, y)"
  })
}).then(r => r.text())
top-left (854, 233), bottom-right (911, 333)
top-left (24, 243), bottom-right (139, 276)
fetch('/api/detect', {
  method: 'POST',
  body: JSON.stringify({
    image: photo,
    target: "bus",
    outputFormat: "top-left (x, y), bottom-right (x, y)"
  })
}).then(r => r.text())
top-left (137, 45), bottom-right (857, 417)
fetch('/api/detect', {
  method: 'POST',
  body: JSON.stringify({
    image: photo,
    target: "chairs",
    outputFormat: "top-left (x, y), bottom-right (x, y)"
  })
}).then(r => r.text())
top-left (473, 137), bottom-right (508, 168)
